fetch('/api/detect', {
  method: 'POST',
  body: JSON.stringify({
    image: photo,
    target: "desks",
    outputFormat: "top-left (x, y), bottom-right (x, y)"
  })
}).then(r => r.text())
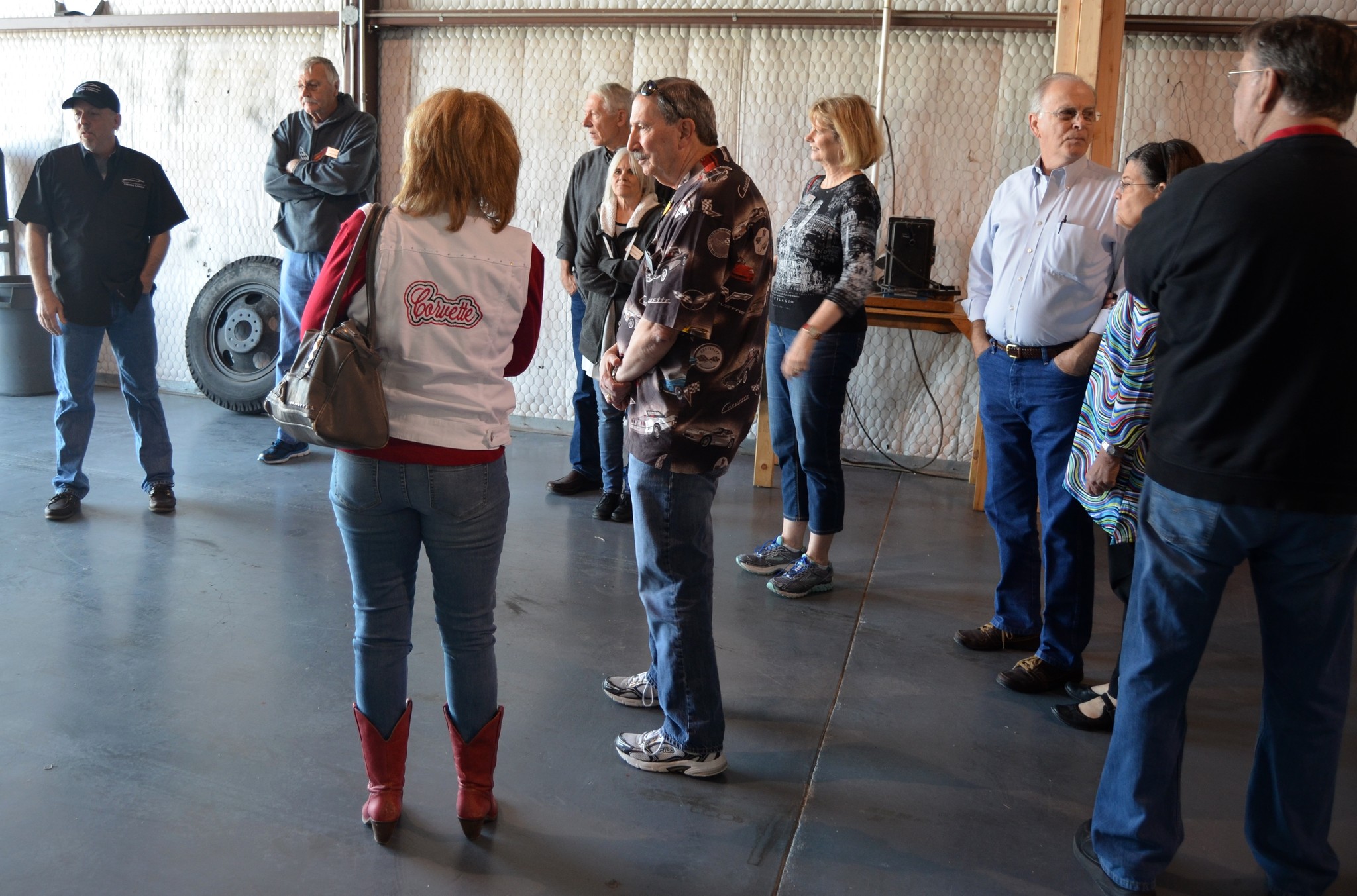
top-left (752, 290), bottom-right (988, 509)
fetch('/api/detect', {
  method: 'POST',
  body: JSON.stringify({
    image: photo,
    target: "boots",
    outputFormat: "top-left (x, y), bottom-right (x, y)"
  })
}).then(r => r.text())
top-left (353, 698), bottom-right (412, 843)
top-left (443, 701), bottom-right (504, 841)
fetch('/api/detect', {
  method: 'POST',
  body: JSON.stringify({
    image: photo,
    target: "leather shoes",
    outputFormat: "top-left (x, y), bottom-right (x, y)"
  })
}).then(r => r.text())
top-left (1065, 681), bottom-right (1102, 702)
top-left (1051, 693), bottom-right (1116, 733)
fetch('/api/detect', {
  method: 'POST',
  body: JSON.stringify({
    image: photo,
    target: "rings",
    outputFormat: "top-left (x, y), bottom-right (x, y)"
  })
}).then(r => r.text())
top-left (607, 393), bottom-right (611, 400)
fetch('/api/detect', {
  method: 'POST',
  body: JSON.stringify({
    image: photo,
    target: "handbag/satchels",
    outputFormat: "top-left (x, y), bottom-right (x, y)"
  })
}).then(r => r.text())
top-left (262, 201), bottom-right (390, 450)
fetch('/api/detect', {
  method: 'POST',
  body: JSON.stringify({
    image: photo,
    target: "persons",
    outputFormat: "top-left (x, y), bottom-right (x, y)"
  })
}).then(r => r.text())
top-left (1072, 14), bottom-right (1357, 896)
top-left (257, 55), bottom-right (377, 461)
top-left (959, 72), bottom-right (1126, 694)
top-left (1060, 138), bottom-right (1203, 719)
top-left (297, 90), bottom-right (544, 842)
top-left (554, 76), bottom-right (772, 776)
top-left (737, 92), bottom-right (884, 596)
top-left (14, 81), bottom-right (189, 519)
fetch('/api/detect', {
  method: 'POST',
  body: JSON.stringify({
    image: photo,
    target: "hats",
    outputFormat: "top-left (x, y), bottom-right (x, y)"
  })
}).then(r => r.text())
top-left (62, 81), bottom-right (120, 113)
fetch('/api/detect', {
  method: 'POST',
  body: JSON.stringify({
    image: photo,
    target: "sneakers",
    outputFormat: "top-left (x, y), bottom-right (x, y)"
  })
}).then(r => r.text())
top-left (257, 441), bottom-right (310, 463)
top-left (953, 622), bottom-right (1041, 652)
top-left (550, 469), bottom-right (603, 495)
top-left (736, 535), bottom-right (807, 575)
top-left (148, 483), bottom-right (176, 512)
top-left (602, 671), bottom-right (660, 707)
top-left (614, 726), bottom-right (728, 776)
top-left (592, 488), bottom-right (621, 520)
top-left (611, 493), bottom-right (633, 523)
top-left (995, 652), bottom-right (1084, 694)
top-left (767, 553), bottom-right (833, 598)
top-left (1073, 818), bottom-right (1156, 896)
top-left (45, 492), bottom-right (81, 519)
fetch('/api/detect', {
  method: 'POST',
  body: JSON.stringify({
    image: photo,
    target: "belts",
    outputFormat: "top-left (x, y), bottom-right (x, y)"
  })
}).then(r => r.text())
top-left (991, 340), bottom-right (1077, 360)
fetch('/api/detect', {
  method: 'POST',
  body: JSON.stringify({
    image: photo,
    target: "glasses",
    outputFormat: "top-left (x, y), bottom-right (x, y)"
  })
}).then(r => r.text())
top-left (1227, 69), bottom-right (1284, 89)
top-left (1118, 180), bottom-right (1166, 193)
top-left (637, 80), bottom-right (684, 119)
top-left (1035, 108), bottom-right (1102, 122)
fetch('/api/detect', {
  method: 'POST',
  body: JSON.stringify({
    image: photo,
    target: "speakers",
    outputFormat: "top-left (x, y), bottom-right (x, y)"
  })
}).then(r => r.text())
top-left (884, 216), bottom-right (934, 294)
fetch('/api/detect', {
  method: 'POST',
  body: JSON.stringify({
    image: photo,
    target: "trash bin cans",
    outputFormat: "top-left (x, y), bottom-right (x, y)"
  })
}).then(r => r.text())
top-left (0, 274), bottom-right (58, 397)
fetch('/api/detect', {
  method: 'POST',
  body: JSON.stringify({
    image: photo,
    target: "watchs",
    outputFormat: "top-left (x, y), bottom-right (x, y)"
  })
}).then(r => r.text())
top-left (611, 366), bottom-right (619, 384)
top-left (1101, 440), bottom-right (1124, 459)
top-left (802, 323), bottom-right (822, 340)
